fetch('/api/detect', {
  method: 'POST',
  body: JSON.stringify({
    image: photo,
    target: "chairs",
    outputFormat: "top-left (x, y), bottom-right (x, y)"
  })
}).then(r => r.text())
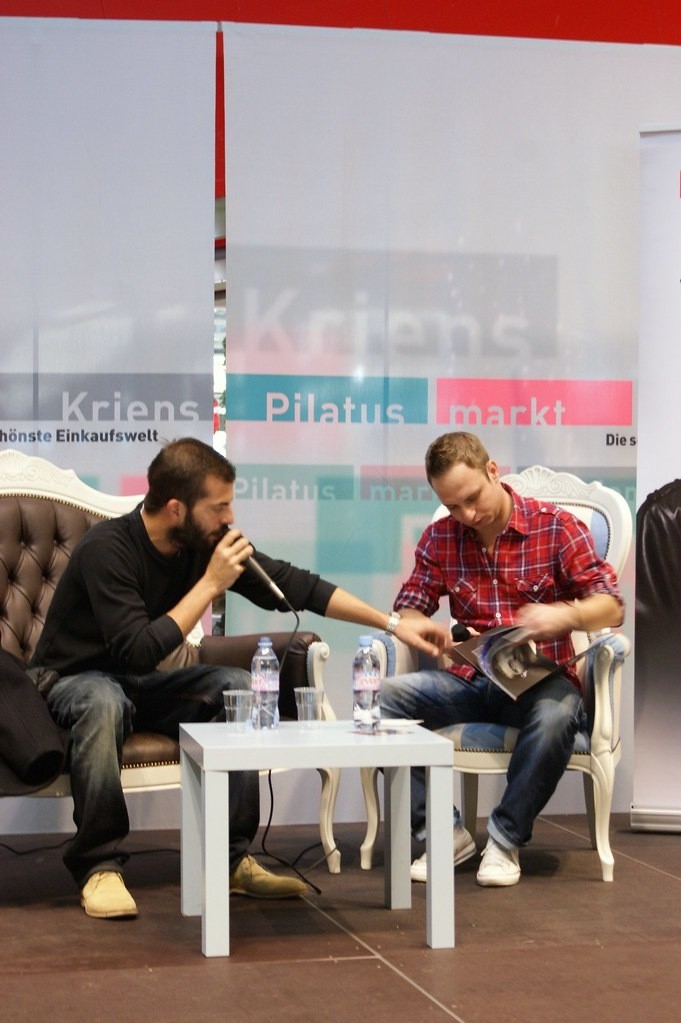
top-left (357, 469), bottom-right (637, 884)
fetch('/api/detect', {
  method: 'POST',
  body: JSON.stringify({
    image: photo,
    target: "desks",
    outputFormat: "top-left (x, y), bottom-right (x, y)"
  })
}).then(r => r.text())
top-left (179, 718), bottom-right (455, 956)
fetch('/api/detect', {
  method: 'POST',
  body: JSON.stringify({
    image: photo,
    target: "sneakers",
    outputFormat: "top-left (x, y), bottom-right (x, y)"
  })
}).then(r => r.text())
top-left (410, 823), bottom-right (476, 882)
top-left (476, 834), bottom-right (522, 886)
top-left (229, 854), bottom-right (307, 899)
top-left (79, 871), bottom-right (138, 918)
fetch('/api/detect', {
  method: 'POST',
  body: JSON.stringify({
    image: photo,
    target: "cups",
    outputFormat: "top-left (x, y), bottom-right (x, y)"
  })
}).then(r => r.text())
top-left (294, 687), bottom-right (324, 729)
top-left (222, 690), bottom-right (253, 732)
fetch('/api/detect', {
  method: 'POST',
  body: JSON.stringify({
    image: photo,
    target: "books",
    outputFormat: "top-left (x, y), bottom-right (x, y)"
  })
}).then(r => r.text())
top-left (453, 623), bottom-right (624, 702)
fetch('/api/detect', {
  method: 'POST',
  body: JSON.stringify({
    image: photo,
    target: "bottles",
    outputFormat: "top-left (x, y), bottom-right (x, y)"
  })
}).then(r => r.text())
top-left (352, 636), bottom-right (382, 734)
top-left (251, 636), bottom-right (280, 730)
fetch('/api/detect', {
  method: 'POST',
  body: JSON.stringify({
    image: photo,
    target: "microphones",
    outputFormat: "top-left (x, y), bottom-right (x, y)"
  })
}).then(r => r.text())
top-left (225, 530), bottom-right (286, 603)
top-left (452, 624), bottom-right (471, 642)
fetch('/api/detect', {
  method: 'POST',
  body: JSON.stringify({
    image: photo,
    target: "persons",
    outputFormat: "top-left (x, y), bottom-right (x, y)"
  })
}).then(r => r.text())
top-left (23, 438), bottom-right (453, 918)
top-left (375, 431), bottom-right (627, 885)
top-left (490, 643), bottom-right (558, 679)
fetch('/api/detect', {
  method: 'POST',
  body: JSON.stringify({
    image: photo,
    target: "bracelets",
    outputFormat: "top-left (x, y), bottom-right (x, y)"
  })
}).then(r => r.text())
top-left (385, 611), bottom-right (402, 635)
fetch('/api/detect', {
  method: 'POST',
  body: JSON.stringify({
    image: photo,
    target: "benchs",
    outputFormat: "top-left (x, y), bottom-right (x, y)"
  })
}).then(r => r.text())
top-left (0, 449), bottom-right (340, 876)
